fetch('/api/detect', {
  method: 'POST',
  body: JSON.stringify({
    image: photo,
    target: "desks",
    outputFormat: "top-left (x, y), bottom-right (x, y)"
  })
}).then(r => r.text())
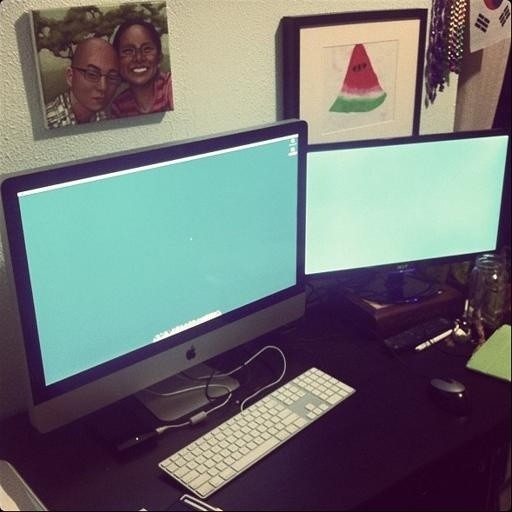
top-left (1, 291), bottom-right (512, 512)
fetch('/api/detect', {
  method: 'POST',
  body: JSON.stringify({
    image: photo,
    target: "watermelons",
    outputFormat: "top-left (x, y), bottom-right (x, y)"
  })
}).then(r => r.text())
top-left (329, 45), bottom-right (387, 112)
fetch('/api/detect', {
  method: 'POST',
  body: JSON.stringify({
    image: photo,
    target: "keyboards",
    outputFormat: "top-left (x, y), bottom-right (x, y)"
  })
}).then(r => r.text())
top-left (158, 367), bottom-right (357, 501)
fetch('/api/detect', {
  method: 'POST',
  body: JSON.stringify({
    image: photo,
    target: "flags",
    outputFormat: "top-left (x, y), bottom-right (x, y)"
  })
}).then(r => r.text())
top-left (466, 0), bottom-right (512, 56)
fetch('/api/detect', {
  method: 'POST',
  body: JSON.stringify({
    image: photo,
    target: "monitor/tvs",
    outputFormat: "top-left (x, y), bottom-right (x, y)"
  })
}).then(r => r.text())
top-left (305, 129), bottom-right (512, 296)
top-left (0, 117), bottom-right (307, 435)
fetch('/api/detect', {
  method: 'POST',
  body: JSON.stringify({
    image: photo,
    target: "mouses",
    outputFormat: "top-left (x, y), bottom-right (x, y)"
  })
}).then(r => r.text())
top-left (430, 377), bottom-right (472, 415)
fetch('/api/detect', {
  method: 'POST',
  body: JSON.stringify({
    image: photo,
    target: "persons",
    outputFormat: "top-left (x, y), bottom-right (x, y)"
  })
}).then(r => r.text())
top-left (46, 36), bottom-right (122, 132)
top-left (109, 20), bottom-right (175, 119)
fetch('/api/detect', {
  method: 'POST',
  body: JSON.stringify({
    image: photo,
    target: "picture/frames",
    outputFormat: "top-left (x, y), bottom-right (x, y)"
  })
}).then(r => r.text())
top-left (281, 8), bottom-right (429, 150)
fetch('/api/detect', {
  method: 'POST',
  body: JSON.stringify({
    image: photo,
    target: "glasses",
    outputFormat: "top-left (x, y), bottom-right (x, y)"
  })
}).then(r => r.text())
top-left (119, 45), bottom-right (161, 57)
top-left (73, 66), bottom-right (123, 87)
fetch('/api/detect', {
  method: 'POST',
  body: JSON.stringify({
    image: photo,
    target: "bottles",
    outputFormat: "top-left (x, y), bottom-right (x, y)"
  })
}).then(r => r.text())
top-left (467, 253), bottom-right (509, 329)
